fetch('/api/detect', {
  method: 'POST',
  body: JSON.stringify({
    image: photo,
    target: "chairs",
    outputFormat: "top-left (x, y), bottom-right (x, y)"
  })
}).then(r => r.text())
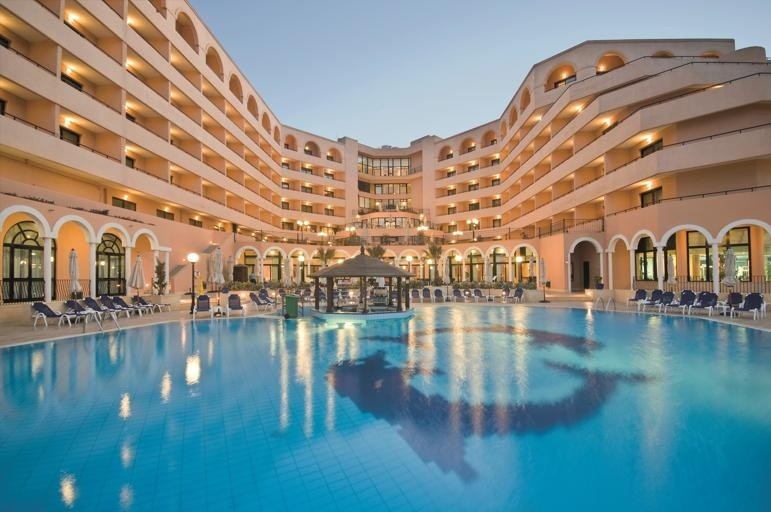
top-left (193, 287), bottom-right (524, 321)
top-left (627, 288), bottom-right (767, 321)
top-left (31, 295), bottom-right (171, 329)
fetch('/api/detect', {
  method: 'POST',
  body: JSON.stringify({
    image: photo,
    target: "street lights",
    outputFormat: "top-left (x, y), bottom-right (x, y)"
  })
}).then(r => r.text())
top-left (405, 256), bottom-right (412, 271)
top-left (296, 221), bottom-right (308, 282)
top-left (455, 255), bottom-right (462, 282)
top-left (515, 257), bottom-right (522, 282)
top-left (418, 226), bottom-right (427, 239)
top-left (466, 218), bottom-right (479, 241)
top-left (187, 253), bottom-right (199, 315)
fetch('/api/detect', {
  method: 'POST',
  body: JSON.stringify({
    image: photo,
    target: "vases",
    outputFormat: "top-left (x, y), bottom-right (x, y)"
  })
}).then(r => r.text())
top-left (595, 284), bottom-right (604, 289)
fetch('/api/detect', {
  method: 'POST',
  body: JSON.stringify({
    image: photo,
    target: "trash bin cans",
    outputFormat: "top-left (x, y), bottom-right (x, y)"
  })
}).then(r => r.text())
top-left (284, 296), bottom-right (298, 319)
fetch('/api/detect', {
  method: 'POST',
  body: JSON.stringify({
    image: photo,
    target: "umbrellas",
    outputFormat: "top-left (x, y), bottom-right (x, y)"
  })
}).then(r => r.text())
top-left (485, 255), bottom-right (493, 301)
top-left (667, 254), bottom-right (676, 292)
top-left (69, 247), bottom-right (83, 310)
top-left (376, 257), bottom-right (386, 287)
top-left (721, 248), bottom-right (736, 310)
top-left (283, 256), bottom-right (292, 289)
top-left (208, 247), bottom-right (225, 303)
top-left (128, 254), bottom-right (145, 305)
top-left (541, 257), bottom-right (547, 301)
top-left (443, 258), bottom-right (450, 296)
